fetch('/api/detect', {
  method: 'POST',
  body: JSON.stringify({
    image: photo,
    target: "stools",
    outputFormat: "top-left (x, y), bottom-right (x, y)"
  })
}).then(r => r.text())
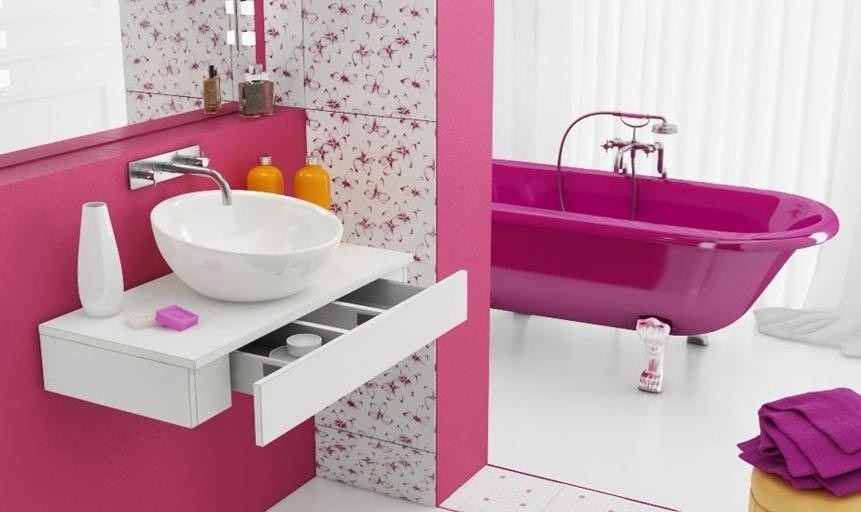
top-left (746, 465), bottom-right (860, 512)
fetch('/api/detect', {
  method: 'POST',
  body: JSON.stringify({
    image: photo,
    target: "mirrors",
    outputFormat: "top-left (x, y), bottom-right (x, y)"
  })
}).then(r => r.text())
top-left (1, 0), bottom-right (267, 168)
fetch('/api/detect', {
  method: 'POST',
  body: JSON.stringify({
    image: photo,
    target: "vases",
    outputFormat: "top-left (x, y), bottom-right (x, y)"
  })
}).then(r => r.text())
top-left (74, 200), bottom-right (126, 319)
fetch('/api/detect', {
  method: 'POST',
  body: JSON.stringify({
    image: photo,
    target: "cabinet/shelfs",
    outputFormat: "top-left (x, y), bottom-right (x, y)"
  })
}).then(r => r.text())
top-left (39, 240), bottom-right (470, 449)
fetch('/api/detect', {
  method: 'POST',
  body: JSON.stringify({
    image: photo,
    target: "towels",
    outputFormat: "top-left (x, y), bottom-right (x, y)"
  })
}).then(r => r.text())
top-left (737, 386), bottom-right (860, 498)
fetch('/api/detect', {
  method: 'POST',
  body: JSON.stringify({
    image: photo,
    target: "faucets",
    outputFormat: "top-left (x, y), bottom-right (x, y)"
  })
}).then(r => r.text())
top-left (152, 160), bottom-right (233, 206)
top-left (613, 142), bottom-right (636, 174)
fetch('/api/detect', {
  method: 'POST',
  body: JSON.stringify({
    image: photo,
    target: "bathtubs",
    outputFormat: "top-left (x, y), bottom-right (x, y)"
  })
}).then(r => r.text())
top-left (490, 158), bottom-right (840, 347)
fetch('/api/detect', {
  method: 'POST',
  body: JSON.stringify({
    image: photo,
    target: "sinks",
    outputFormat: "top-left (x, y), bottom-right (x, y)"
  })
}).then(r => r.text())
top-left (148, 189), bottom-right (344, 303)
